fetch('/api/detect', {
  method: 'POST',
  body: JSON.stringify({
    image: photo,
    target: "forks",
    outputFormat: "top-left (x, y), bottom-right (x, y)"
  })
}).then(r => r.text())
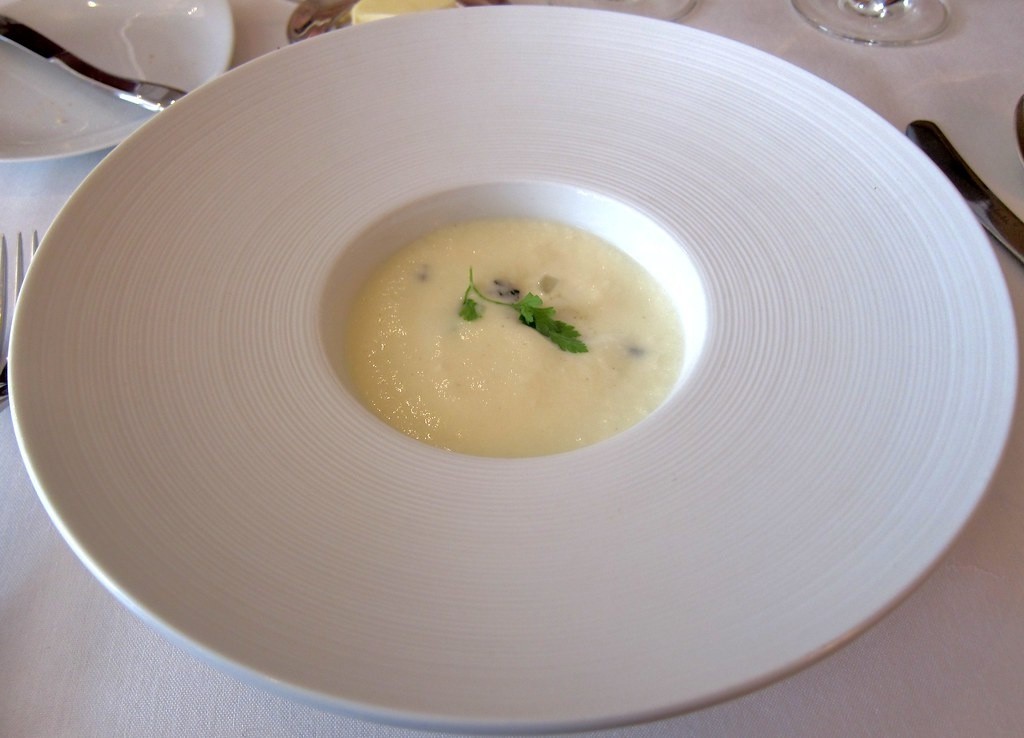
top-left (0, 231), bottom-right (40, 405)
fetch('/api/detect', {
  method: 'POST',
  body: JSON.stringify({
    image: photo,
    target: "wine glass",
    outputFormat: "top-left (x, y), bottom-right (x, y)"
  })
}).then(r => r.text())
top-left (547, 0), bottom-right (698, 21)
top-left (791, 0), bottom-right (951, 44)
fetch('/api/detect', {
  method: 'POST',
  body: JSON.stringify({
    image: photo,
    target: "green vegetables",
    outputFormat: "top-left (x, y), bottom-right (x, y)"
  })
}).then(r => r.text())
top-left (457, 266), bottom-right (589, 355)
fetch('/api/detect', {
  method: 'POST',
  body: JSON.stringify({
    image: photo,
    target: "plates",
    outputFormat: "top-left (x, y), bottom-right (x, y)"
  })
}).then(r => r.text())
top-left (8, 6), bottom-right (1020, 734)
top-left (0, 0), bottom-right (235, 161)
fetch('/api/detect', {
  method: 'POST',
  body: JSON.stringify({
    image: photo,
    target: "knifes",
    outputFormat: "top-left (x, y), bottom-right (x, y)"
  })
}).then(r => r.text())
top-left (907, 120), bottom-right (1024, 261)
top-left (0, 15), bottom-right (188, 113)
